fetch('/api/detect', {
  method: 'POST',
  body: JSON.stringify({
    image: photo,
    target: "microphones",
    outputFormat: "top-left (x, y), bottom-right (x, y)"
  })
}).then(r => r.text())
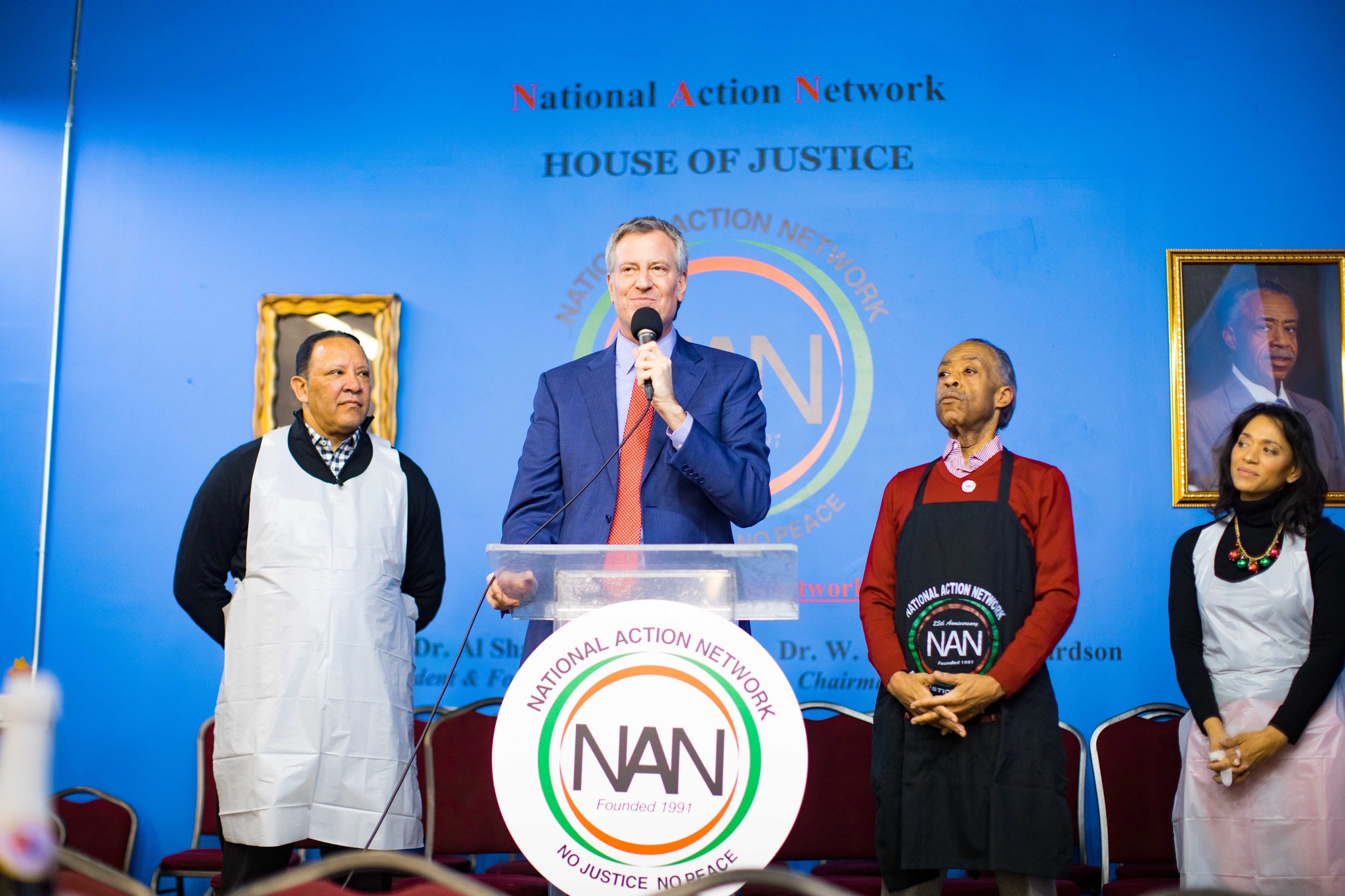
top-left (630, 307), bottom-right (663, 400)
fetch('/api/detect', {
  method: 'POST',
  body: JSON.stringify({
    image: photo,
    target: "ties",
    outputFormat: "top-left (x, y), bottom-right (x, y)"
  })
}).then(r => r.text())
top-left (603, 376), bottom-right (654, 596)
top-left (1275, 399), bottom-right (1288, 408)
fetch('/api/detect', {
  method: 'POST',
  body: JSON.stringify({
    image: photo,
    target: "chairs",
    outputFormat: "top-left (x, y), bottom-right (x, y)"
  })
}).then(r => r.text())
top-left (424, 693), bottom-right (549, 896)
top-left (229, 848), bottom-right (514, 896)
top-left (147, 715), bottom-right (307, 896)
top-left (1090, 703), bottom-right (1191, 896)
top-left (413, 706), bottom-right (478, 875)
top-left (965, 721), bottom-right (1101, 896)
top-left (53, 843), bottom-right (157, 896)
top-left (647, 700), bottom-right (884, 896)
top-left (483, 859), bottom-right (544, 877)
top-left (45, 786), bottom-right (138, 877)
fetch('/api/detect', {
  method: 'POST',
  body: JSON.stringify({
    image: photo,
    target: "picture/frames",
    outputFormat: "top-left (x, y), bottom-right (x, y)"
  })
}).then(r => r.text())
top-left (252, 289), bottom-right (403, 448)
top-left (1166, 246), bottom-right (1345, 509)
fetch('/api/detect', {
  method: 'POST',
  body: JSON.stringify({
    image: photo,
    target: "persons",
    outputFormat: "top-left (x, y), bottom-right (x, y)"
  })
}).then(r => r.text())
top-left (1186, 280), bottom-right (1345, 493)
top-left (173, 330), bottom-right (446, 896)
top-left (859, 339), bottom-right (1080, 896)
top-left (1167, 402), bottom-right (1345, 896)
top-left (487, 217), bottom-right (772, 670)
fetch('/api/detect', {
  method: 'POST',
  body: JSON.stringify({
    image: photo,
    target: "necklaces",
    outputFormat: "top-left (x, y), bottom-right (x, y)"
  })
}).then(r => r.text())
top-left (1228, 516), bottom-right (1284, 573)
